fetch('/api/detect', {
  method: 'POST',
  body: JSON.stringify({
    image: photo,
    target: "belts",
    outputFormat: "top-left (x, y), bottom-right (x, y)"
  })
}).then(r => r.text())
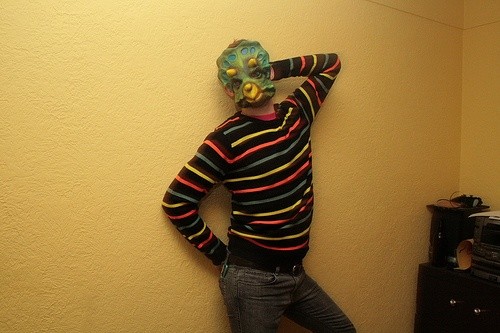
top-left (227, 254), bottom-right (303, 276)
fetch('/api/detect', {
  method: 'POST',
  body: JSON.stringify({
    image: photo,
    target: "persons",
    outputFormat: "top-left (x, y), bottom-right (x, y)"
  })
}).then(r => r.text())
top-left (162, 35), bottom-right (358, 332)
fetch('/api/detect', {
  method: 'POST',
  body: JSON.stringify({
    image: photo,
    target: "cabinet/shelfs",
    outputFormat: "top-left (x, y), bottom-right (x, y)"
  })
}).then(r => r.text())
top-left (415, 264), bottom-right (500, 332)
